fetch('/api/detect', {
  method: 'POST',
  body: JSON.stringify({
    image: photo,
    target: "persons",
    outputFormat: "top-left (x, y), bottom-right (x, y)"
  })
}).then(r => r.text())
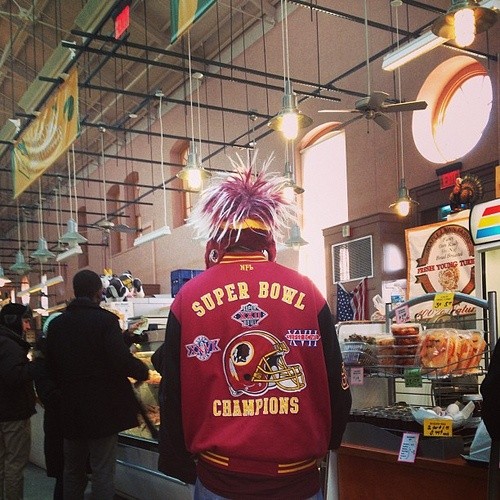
top-left (46, 269), bottom-right (150, 500)
top-left (0, 302), bottom-right (39, 500)
top-left (152, 150), bottom-right (354, 500)
top-left (479, 336), bottom-right (500, 500)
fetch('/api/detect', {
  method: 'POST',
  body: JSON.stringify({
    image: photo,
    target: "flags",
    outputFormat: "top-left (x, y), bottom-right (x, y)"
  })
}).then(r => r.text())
top-left (170, 0), bottom-right (217, 44)
top-left (9, 65), bottom-right (84, 201)
top-left (336, 275), bottom-right (371, 321)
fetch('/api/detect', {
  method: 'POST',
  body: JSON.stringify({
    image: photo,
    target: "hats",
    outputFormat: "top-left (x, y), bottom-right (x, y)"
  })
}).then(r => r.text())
top-left (0, 303), bottom-right (33, 342)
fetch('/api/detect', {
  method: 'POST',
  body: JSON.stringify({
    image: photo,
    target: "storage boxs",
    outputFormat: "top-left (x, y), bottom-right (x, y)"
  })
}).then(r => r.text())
top-left (170, 268), bottom-right (206, 297)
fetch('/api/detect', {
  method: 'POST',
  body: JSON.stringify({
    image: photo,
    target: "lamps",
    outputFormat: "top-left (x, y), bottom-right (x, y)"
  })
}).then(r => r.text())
top-left (129, 92), bottom-right (173, 246)
top-left (389, 2), bottom-right (420, 217)
top-left (176, 31), bottom-right (212, 189)
top-left (1, 125), bottom-right (116, 289)
top-left (267, 1), bottom-right (312, 142)
top-left (431, 0), bottom-right (499, 49)
top-left (275, 142), bottom-right (306, 202)
